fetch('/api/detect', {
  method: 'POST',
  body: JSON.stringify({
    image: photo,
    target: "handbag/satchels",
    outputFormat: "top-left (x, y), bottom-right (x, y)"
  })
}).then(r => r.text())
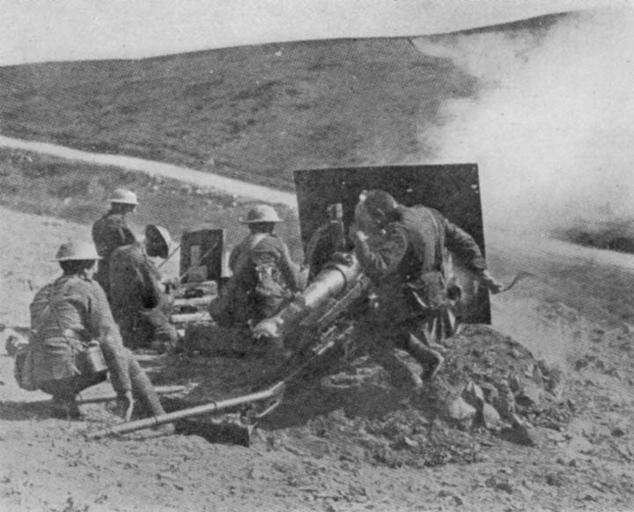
top-left (210, 278), bottom-right (246, 327)
top-left (14, 345), bottom-right (35, 391)
top-left (399, 263), bottom-right (463, 326)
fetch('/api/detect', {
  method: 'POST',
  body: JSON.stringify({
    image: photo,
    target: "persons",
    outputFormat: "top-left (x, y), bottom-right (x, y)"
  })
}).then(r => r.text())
top-left (13, 238), bottom-right (175, 433)
top-left (109, 224), bottom-right (174, 353)
top-left (91, 188), bottom-right (137, 300)
top-left (227, 204), bottom-right (312, 330)
top-left (348, 188), bottom-right (506, 388)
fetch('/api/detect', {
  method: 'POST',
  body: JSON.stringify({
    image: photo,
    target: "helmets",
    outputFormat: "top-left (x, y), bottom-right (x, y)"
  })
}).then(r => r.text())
top-left (238, 204), bottom-right (285, 226)
top-left (51, 239), bottom-right (105, 262)
top-left (146, 223), bottom-right (172, 258)
top-left (107, 188), bottom-right (139, 206)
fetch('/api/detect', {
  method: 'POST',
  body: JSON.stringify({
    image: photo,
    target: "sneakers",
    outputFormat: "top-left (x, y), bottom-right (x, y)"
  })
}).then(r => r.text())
top-left (50, 398), bottom-right (85, 415)
top-left (423, 350), bottom-right (444, 381)
